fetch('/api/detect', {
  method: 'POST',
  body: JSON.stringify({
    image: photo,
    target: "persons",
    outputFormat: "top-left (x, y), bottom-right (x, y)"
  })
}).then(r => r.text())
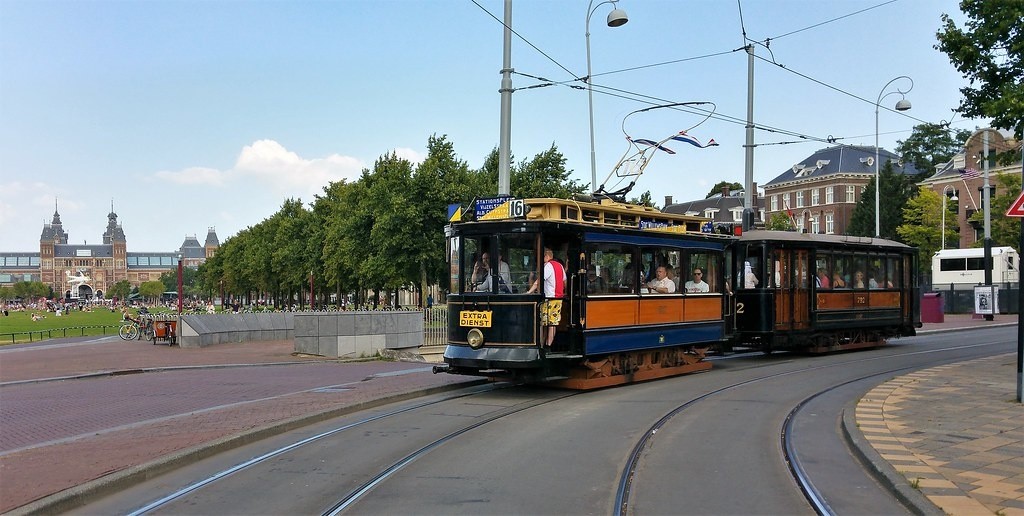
top-left (587, 265), bottom-right (619, 293)
top-left (644, 266), bottom-right (675, 294)
top-left (0, 297), bottom-right (92, 322)
top-left (229, 300), bottom-right (241, 313)
top-left (472, 250), bottom-right (512, 294)
top-left (166, 297), bottom-right (200, 308)
top-left (737, 261), bottom-right (759, 288)
top-left (815, 267), bottom-right (893, 288)
top-left (103, 299), bottom-right (132, 338)
top-left (625, 253), bottom-right (680, 292)
top-left (527, 247), bottom-right (567, 353)
top-left (206, 301), bottom-right (215, 313)
top-left (684, 268), bottom-right (709, 292)
top-left (427, 294), bottom-right (433, 308)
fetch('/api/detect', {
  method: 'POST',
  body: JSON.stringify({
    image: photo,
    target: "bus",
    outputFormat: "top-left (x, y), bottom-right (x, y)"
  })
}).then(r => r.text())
top-left (432, 101), bottom-right (924, 392)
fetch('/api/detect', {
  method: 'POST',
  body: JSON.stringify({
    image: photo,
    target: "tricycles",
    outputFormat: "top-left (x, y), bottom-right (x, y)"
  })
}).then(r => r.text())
top-left (153, 320), bottom-right (178, 346)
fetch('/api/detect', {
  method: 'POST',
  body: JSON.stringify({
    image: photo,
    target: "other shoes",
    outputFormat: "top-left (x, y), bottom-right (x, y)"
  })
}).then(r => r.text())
top-left (544, 345), bottom-right (552, 353)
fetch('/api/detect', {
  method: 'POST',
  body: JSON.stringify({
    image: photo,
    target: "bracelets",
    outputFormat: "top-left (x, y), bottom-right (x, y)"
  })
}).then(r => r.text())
top-left (653, 286), bottom-right (657, 290)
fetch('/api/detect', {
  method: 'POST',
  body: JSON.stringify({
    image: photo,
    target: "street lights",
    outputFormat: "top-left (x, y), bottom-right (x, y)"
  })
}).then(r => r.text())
top-left (942, 184), bottom-right (959, 248)
top-left (587, 0), bottom-right (627, 189)
top-left (873, 76), bottom-right (915, 234)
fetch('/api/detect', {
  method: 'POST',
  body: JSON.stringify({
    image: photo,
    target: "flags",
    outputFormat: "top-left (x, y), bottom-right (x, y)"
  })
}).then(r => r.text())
top-left (632, 135), bottom-right (719, 155)
top-left (787, 207), bottom-right (792, 217)
top-left (958, 168), bottom-right (979, 180)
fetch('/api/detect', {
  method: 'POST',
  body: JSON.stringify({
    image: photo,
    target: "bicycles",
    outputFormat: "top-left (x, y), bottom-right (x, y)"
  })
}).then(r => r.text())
top-left (118, 309), bottom-right (155, 341)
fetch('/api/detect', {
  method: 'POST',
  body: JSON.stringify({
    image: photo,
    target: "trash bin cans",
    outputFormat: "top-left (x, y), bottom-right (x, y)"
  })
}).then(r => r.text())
top-left (921, 293), bottom-right (944, 323)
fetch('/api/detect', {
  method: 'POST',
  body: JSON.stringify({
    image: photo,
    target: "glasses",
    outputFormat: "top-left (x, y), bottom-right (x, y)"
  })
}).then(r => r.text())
top-left (694, 273), bottom-right (701, 275)
